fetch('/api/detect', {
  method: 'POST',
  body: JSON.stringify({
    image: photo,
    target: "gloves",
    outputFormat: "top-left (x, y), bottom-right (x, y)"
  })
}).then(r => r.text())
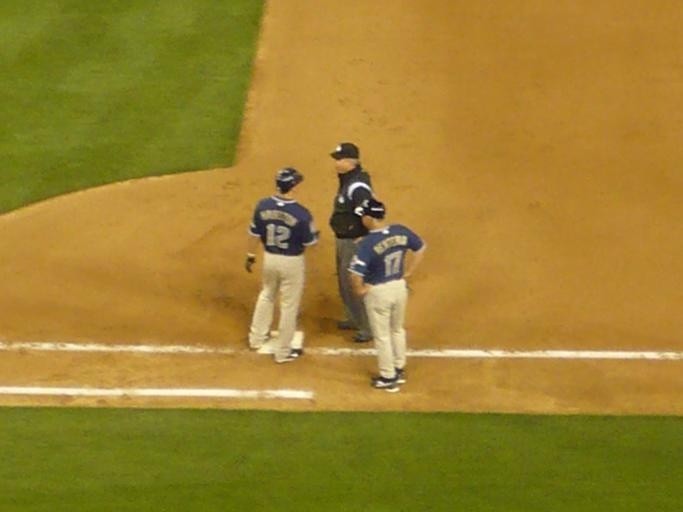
top-left (245, 253), bottom-right (256, 273)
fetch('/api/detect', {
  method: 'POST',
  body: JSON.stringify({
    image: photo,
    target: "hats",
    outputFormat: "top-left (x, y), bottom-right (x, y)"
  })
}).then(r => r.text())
top-left (329, 143), bottom-right (358, 159)
top-left (275, 349), bottom-right (300, 363)
top-left (275, 168), bottom-right (302, 193)
top-left (353, 198), bottom-right (385, 220)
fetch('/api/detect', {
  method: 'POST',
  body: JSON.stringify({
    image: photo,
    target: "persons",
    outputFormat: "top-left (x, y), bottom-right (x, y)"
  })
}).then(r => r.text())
top-left (329, 142), bottom-right (376, 342)
top-left (347, 199), bottom-right (426, 393)
top-left (244, 167), bottom-right (321, 363)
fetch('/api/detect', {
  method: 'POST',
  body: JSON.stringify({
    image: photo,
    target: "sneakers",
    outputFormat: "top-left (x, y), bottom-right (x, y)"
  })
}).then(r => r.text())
top-left (395, 369), bottom-right (405, 380)
top-left (337, 320), bottom-right (352, 328)
top-left (371, 377), bottom-right (393, 389)
top-left (354, 330), bottom-right (371, 342)
